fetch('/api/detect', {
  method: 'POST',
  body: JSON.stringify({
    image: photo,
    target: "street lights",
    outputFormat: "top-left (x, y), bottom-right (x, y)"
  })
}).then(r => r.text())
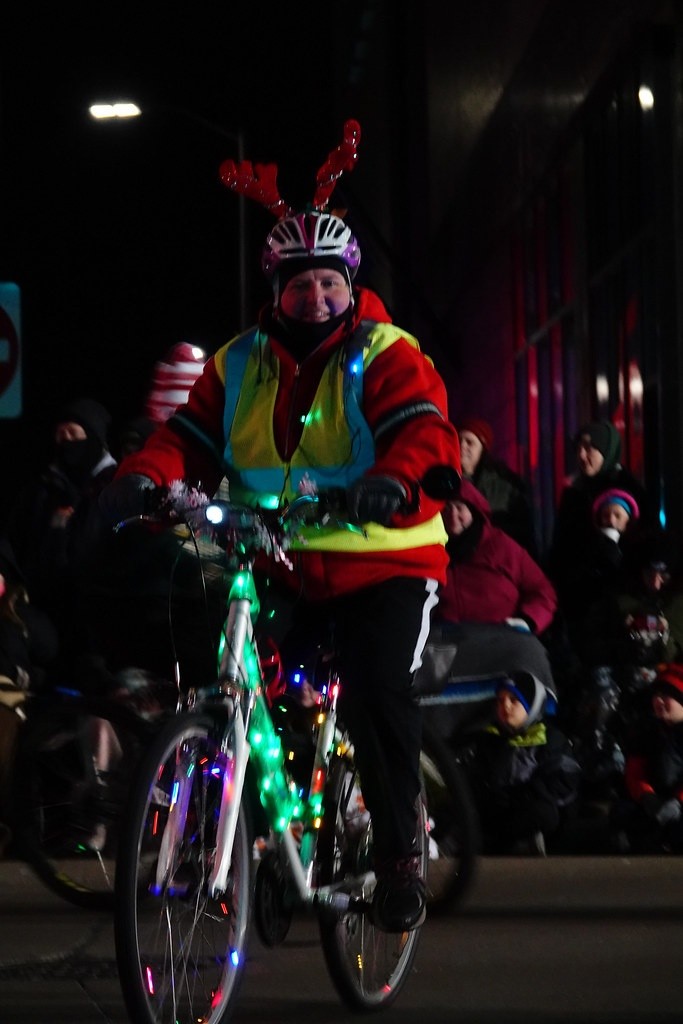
top-left (87, 93), bottom-right (247, 335)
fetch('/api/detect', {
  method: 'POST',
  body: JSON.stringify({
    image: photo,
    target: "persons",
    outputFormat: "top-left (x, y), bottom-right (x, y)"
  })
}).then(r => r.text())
top-left (432, 418), bottom-right (683, 856)
top-left (104, 212), bottom-right (459, 931)
top-left (0, 342), bottom-right (322, 854)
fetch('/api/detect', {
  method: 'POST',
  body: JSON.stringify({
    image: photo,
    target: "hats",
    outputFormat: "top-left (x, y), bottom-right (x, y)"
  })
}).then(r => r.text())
top-left (579, 420), bottom-right (611, 460)
top-left (458, 416), bottom-right (501, 454)
top-left (141, 342), bottom-right (208, 423)
top-left (607, 496), bottom-right (633, 518)
top-left (654, 662), bottom-right (683, 704)
top-left (497, 670), bottom-right (534, 715)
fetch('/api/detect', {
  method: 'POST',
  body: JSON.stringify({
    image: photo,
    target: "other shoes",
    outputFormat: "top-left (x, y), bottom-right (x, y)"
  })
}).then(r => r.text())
top-left (368, 863), bottom-right (434, 933)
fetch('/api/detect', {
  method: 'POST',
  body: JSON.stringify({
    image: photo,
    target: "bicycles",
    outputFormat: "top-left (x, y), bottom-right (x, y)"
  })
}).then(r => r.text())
top-left (4, 463), bottom-right (482, 1024)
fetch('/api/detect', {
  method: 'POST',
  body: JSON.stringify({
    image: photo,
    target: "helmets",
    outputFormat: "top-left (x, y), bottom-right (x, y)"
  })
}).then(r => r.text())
top-left (261, 209), bottom-right (365, 283)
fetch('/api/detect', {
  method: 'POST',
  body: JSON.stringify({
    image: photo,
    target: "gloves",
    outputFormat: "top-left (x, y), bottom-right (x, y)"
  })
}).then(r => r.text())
top-left (94, 473), bottom-right (157, 522)
top-left (344, 475), bottom-right (409, 527)
